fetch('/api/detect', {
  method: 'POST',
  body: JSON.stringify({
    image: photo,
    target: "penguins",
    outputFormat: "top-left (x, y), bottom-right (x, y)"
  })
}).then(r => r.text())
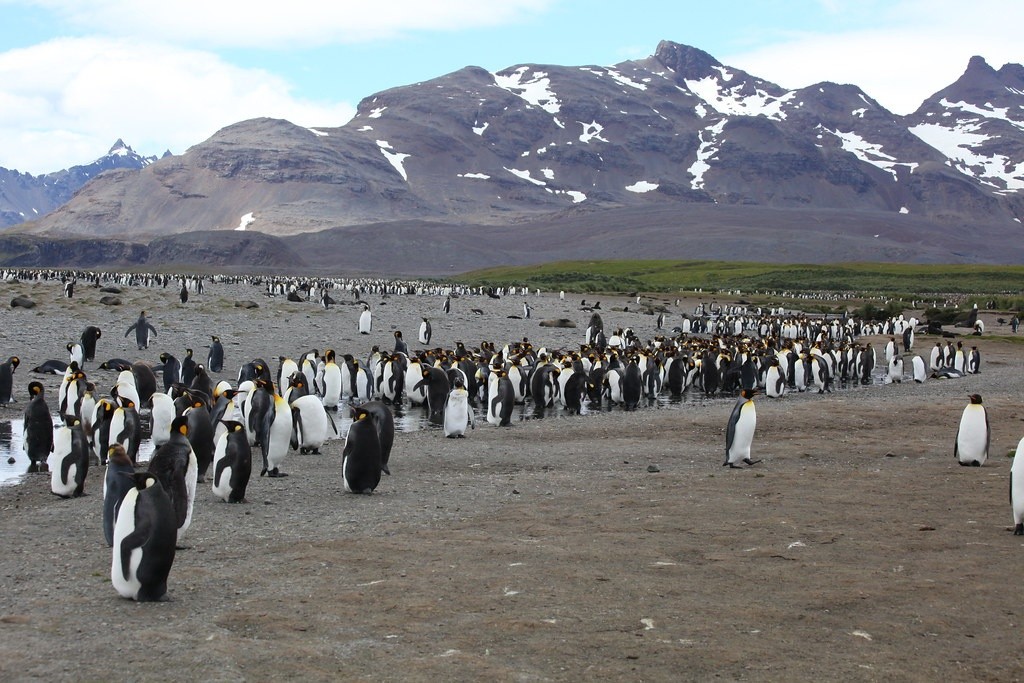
top-left (246, 378), bottom-right (293, 479)
top-left (585, 312), bottom-right (604, 346)
top-left (634, 284), bottom-right (1022, 336)
top-left (1006, 436), bottom-right (1024, 537)
top-left (212, 419), bottom-right (254, 505)
top-left (125, 310), bottom-right (158, 351)
top-left (0, 325), bottom-right (977, 457)
top-left (951, 393), bottom-right (992, 468)
top-left (722, 387), bottom-right (763, 470)
top-left (355, 302), bottom-right (373, 336)
top-left (109, 470), bottom-right (178, 602)
top-left (417, 313), bottom-right (432, 346)
top-left (0, 263), bottom-right (630, 318)
top-left (339, 402), bottom-right (391, 499)
top-left (18, 380), bottom-right (56, 474)
top-left (49, 409), bottom-right (89, 502)
top-left (101, 442), bottom-right (137, 550)
top-left (146, 416), bottom-right (198, 551)
